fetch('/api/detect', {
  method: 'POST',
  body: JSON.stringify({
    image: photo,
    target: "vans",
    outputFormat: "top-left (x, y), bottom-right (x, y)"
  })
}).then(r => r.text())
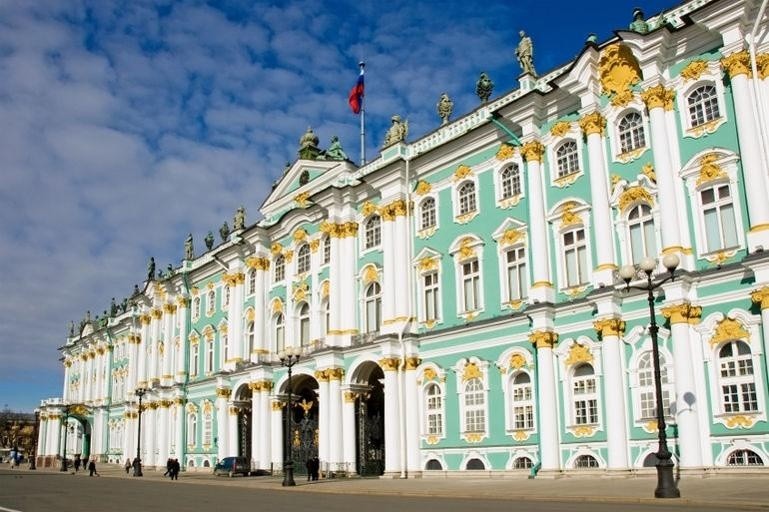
top-left (212, 456), bottom-right (252, 477)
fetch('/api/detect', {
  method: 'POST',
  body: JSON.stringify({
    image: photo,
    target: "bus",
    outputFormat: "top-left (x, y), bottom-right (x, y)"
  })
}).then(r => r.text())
top-left (0, 447), bottom-right (27, 464)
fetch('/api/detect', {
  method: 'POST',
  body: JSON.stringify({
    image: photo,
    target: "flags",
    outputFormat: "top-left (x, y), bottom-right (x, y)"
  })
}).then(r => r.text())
top-left (347, 69), bottom-right (366, 117)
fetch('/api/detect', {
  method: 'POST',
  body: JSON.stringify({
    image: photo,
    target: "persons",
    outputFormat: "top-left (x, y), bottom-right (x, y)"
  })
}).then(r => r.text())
top-left (124, 458), bottom-right (132, 474)
top-left (182, 231), bottom-right (193, 259)
top-left (305, 455), bottom-right (315, 481)
top-left (312, 457), bottom-right (317, 480)
top-left (312, 454), bottom-right (320, 479)
top-left (73, 455), bottom-right (81, 472)
top-left (163, 457), bottom-right (180, 480)
top-left (274, 159), bottom-right (292, 185)
top-left (132, 457), bottom-right (137, 470)
top-left (232, 205), bottom-right (244, 228)
top-left (87, 459), bottom-right (96, 477)
top-left (515, 30), bottom-right (538, 77)
top-left (9, 455), bottom-right (16, 469)
top-left (316, 134), bottom-right (350, 161)
top-left (381, 113), bottom-right (404, 144)
top-left (82, 455), bottom-right (88, 470)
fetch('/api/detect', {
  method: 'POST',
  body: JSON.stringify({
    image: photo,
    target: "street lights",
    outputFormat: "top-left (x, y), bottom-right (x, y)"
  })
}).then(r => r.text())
top-left (274, 343), bottom-right (303, 488)
top-left (59, 400), bottom-right (72, 471)
top-left (616, 251), bottom-right (686, 498)
top-left (131, 382), bottom-right (148, 477)
top-left (29, 406), bottom-right (41, 469)
